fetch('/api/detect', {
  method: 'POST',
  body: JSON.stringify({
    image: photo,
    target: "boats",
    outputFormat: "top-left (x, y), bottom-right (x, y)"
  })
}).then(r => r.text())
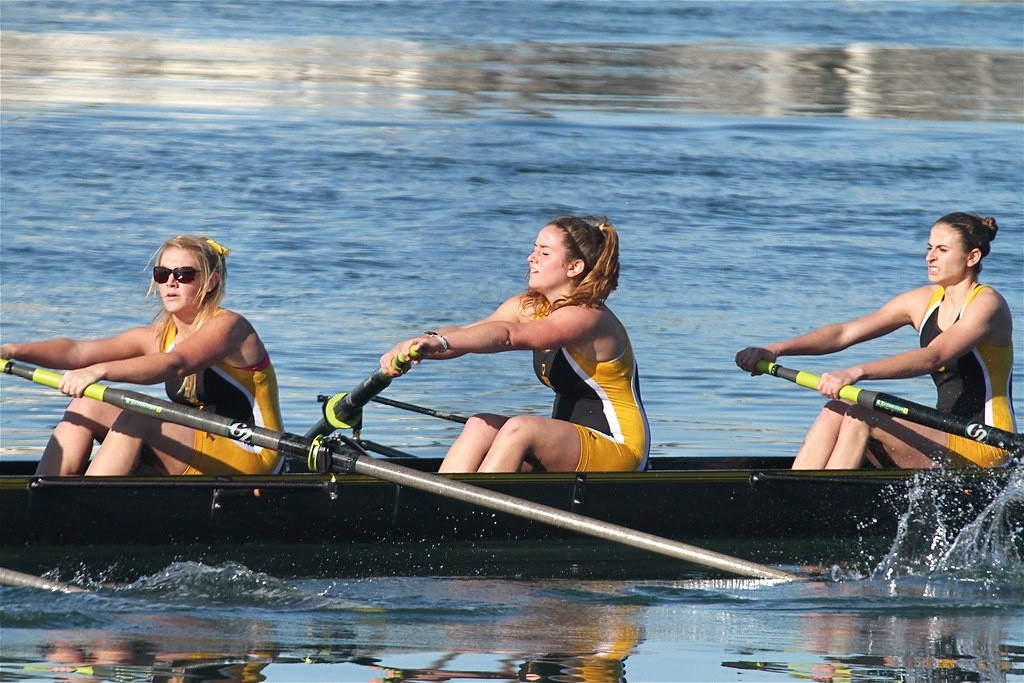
top-left (0, 455), bottom-right (1023, 586)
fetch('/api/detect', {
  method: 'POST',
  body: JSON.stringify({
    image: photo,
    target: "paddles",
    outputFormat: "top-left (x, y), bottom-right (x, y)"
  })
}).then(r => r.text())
top-left (304, 344), bottom-right (422, 439)
top-left (1, 356), bottom-right (792, 582)
top-left (755, 359), bottom-right (1024, 451)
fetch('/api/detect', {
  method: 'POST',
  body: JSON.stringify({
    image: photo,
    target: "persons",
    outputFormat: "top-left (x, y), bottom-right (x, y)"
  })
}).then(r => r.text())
top-left (381, 216), bottom-right (650, 474)
top-left (0, 235), bottom-right (286, 479)
top-left (736, 211), bottom-right (1017, 472)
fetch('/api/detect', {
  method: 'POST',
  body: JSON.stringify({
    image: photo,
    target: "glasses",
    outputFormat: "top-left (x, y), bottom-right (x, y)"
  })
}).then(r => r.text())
top-left (153, 267), bottom-right (202, 284)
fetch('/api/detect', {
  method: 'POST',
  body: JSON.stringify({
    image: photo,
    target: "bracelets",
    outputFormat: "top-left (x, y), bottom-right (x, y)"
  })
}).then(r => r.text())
top-left (425, 331), bottom-right (436, 337)
top-left (432, 334), bottom-right (447, 352)
